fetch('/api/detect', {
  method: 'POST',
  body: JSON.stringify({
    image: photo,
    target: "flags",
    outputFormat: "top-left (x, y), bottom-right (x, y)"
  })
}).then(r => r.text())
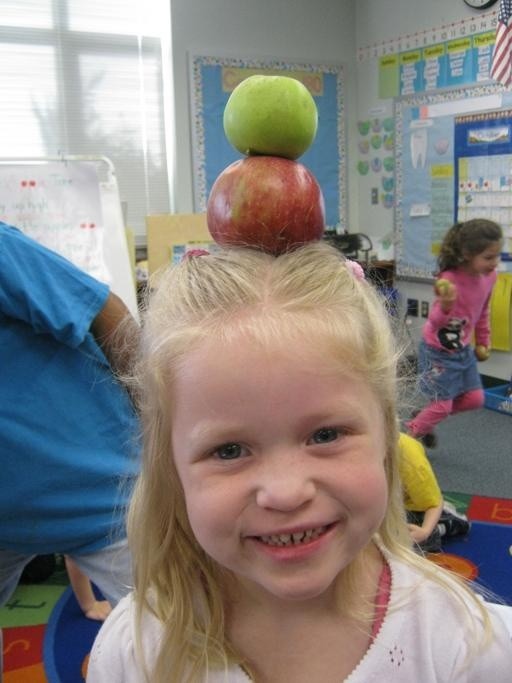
top-left (489, 0), bottom-right (511, 87)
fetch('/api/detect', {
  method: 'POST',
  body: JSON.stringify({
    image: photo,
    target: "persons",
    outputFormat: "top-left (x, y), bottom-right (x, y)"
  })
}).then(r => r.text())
top-left (401, 220), bottom-right (505, 449)
top-left (86, 242), bottom-right (511, 683)
top-left (63, 551), bottom-right (115, 621)
top-left (1, 219), bottom-right (151, 611)
top-left (391, 431), bottom-right (471, 545)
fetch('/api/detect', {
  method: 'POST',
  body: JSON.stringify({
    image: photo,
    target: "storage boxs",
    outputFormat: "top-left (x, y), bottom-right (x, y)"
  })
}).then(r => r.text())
top-left (483, 384), bottom-right (512, 415)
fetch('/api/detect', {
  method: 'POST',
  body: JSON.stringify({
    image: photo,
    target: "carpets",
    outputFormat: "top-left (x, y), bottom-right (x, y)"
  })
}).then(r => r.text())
top-left (1, 492), bottom-right (512, 683)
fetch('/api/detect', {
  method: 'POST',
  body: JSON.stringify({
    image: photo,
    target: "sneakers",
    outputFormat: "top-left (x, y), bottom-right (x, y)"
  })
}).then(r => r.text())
top-left (436, 499), bottom-right (472, 538)
top-left (412, 407), bottom-right (439, 449)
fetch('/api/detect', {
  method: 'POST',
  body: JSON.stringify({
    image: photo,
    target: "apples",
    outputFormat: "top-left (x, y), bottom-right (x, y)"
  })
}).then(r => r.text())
top-left (435, 277), bottom-right (451, 294)
top-left (209, 156), bottom-right (325, 247)
top-left (222, 76), bottom-right (320, 158)
top-left (474, 345), bottom-right (490, 361)
